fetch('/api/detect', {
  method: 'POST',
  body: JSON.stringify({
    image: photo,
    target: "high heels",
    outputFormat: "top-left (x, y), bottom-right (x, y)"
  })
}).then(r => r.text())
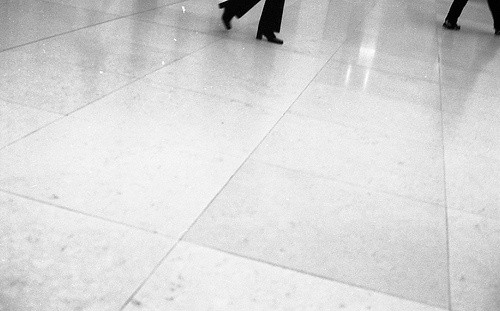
top-left (256, 31), bottom-right (283, 44)
top-left (219, 1), bottom-right (234, 29)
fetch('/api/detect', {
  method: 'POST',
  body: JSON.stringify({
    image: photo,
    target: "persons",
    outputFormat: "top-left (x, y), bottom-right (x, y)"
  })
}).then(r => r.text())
top-left (443, 0), bottom-right (500, 36)
top-left (218, 0), bottom-right (285, 45)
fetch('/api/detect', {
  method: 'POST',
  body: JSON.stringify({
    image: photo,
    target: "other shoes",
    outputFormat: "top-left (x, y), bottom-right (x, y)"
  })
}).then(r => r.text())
top-left (494, 30), bottom-right (500, 35)
top-left (442, 23), bottom-right (460, 29)
